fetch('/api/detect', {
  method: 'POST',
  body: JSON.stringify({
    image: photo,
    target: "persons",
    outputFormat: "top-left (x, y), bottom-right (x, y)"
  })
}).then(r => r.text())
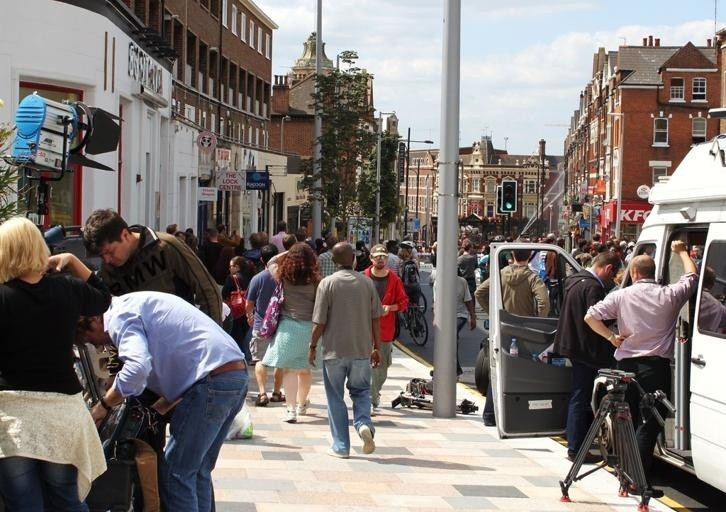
top-left (360, 244), bottom-right (395, 416)
top-left (475, 238), bottom-right (549, 425)
top-left (584, 240), bottom-right (697, 499)
top-left (553, 250), bottom-right (622, 463)
top-left (267, 242), bottom-right (322, 423)
top-left (1, 209), bottom-right (251, 512)
top-left (168, 220), bottom-right (420, 405)
top-left (308, 242), bottom-right (383, 458)
top-left (698, 266), bottom-right (726, 335)
top-left (430, 234), bottom-right (708, 383)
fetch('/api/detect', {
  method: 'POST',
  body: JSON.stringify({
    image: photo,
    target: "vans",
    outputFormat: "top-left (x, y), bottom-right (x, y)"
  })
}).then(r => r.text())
top-left (487, 134), bottom-right (726, 496)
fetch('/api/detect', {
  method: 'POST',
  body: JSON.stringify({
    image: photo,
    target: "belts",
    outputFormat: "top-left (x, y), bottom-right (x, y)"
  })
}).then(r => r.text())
top-left (210, 359), bottom-right (249, 376)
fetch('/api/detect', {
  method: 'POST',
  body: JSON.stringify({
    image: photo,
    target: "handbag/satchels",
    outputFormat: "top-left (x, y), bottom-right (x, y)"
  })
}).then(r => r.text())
top-left (257, 297), bottom-right (283, 338)
top-left (230, 291), bottom-right (247, 318)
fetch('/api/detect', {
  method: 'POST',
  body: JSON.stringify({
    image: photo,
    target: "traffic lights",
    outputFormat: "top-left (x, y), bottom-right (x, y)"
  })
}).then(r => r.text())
top-left (498, 187), bottom-right (508, 215)
top-left (503, 182), bottom-right (515, 212)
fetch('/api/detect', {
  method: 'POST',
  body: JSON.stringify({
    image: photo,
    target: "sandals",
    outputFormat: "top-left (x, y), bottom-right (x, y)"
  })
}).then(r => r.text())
top-left (256, 393), bottom-right (268, 405)
top-left (270, 391), bottom-right (285, 401)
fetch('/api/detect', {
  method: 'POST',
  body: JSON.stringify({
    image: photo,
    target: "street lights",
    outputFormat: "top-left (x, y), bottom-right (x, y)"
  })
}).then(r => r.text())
top-left (395, 139), bottom-right (434, 242)
top-left (537, 136), bottom-right (545, 237)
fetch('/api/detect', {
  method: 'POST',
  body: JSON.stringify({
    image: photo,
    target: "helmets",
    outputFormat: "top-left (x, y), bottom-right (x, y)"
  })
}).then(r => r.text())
top-left (402, 241), bottom-right (414, 249)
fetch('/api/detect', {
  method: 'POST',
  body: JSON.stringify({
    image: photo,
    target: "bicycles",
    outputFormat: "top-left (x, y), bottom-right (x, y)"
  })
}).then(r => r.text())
top-left (394, 302), bottom-right (427, 346)
top-left (403, 286), bottom-right (427, 312)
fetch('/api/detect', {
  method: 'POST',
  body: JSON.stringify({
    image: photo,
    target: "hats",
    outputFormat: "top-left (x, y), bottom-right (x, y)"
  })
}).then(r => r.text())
top-left (370, 243), bottom-right (389, 258)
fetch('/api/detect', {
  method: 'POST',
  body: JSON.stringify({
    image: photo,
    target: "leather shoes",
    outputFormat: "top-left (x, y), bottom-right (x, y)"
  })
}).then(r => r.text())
top-left (571, 453), bottom-right (602, 463)
top-left (631, 488), bottom-right (663, 498)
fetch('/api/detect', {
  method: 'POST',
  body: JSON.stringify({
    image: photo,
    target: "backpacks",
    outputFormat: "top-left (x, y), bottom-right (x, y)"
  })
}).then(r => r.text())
top-left (401, 260), bottom-right (420, 287)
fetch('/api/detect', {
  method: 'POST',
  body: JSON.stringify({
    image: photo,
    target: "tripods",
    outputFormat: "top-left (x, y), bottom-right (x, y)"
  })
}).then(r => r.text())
top-left (559, 369), bottom-right (676, 512)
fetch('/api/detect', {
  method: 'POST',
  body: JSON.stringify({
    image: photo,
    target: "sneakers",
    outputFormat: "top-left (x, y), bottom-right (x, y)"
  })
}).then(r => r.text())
top-left (297, 399), bottom-right (310, 415)
top-left (359, 425), bottom-right (374, 453)
top-left (281, 410), bottom-right (297, 422)
top-left (327, 447), bottom-right (350, 458)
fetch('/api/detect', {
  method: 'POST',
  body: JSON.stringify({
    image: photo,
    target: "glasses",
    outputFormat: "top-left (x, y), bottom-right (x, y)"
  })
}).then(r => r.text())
top-left (375, 256), bottom-right (385, 261)
